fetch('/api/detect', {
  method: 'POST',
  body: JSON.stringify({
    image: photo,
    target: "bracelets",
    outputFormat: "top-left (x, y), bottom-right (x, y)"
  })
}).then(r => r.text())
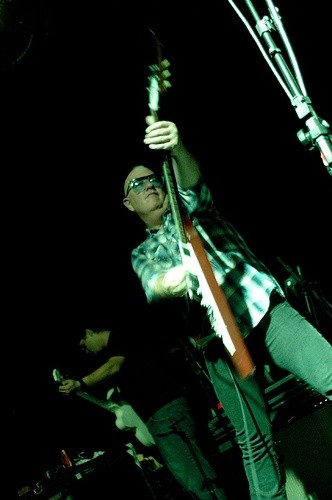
top-left (78, 378), bottom-right (87, 393)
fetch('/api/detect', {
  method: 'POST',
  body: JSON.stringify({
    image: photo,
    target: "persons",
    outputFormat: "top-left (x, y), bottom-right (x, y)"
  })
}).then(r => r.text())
top-left (57, 313), bottom-right (227, 499)
top-left (111, 115), bottom-right (332, 499)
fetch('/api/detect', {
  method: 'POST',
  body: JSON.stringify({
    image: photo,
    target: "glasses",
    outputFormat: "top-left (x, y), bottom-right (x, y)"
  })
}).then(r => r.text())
top-left (125, 173), bottom-right (160, 215)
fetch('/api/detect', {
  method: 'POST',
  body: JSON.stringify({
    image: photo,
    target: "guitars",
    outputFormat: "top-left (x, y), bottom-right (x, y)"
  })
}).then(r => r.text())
top-left (52, 368), bottom-right (157, 446)
top-left (147, 59), bottom-right (257, 380)
